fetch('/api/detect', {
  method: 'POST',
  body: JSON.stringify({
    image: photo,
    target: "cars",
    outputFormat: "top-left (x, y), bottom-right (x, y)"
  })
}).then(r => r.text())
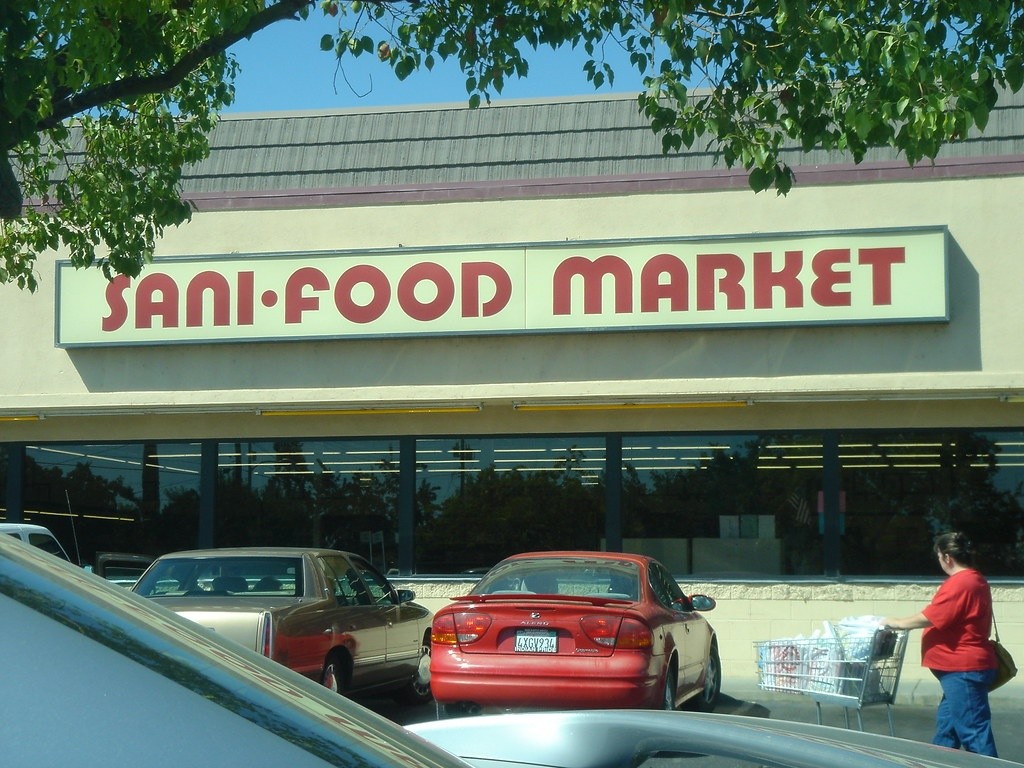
top-left (0, 532), bottom-right (470, 768)
top-left (429, 550), bottom-right (723, 721)
top-left (0, 523), bottom-right (94, 574)
top-left (91, 546), bottom-right (436, 703)
top-left (403, 708), bottom-right (1024, 768)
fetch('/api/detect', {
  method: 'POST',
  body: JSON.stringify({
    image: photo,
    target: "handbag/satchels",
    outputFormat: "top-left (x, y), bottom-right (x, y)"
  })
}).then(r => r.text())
top-left (759, 614), bottom-right (897, 700)
top-left (984, 607), bottom-right (1018, 693)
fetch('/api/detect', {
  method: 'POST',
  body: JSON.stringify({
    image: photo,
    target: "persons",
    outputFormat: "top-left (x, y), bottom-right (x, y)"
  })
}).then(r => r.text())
top-left (881, 531), bottom-right (1001, 758)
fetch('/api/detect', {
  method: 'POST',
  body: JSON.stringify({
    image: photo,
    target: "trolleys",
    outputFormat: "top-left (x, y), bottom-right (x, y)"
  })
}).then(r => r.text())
top-left (752, 625), bottom-right (908, 737)
